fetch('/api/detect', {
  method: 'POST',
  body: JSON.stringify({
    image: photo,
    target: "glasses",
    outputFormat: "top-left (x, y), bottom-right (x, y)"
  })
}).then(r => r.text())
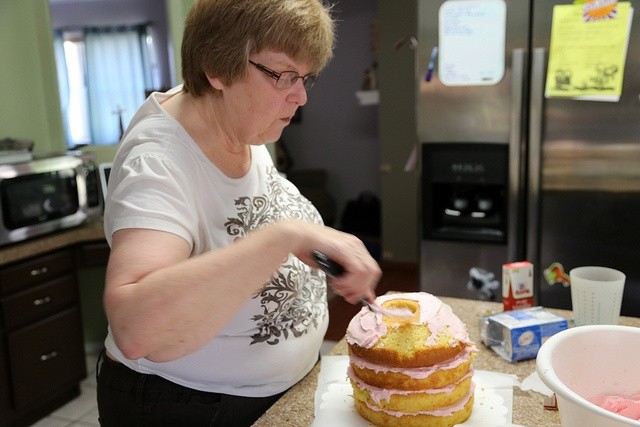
top-left (248, 60), bottom-right (319, 90)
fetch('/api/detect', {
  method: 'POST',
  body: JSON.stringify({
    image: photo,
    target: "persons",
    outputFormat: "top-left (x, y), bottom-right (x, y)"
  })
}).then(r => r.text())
top-left (96, 0), bottom-right (384, 427)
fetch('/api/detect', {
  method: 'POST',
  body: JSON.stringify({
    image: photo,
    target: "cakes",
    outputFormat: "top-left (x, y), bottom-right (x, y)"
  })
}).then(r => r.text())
top-left (344, 290), bottom-right (479, 425)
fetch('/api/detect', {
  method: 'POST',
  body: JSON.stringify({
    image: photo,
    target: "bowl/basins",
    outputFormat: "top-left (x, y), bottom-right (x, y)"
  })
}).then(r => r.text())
top-left (536, 324), bottom-right (640, 427)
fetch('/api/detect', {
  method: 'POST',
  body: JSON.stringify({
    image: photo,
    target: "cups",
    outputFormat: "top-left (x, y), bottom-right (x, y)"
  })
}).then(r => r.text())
top-left (569, 267), bottom-right (626, 327)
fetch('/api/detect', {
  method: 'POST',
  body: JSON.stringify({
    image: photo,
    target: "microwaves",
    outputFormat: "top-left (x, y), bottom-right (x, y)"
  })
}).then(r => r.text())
top-left (0, 146), bottom-right (89, 247)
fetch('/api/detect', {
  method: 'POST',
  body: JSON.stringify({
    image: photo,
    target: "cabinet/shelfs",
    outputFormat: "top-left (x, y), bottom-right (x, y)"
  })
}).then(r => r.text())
top-left (0, 247), bottom-right (87, 426)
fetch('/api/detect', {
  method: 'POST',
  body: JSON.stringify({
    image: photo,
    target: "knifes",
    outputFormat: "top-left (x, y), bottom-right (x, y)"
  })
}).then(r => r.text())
top-left (311, 250), bottom-right (402, 317)
